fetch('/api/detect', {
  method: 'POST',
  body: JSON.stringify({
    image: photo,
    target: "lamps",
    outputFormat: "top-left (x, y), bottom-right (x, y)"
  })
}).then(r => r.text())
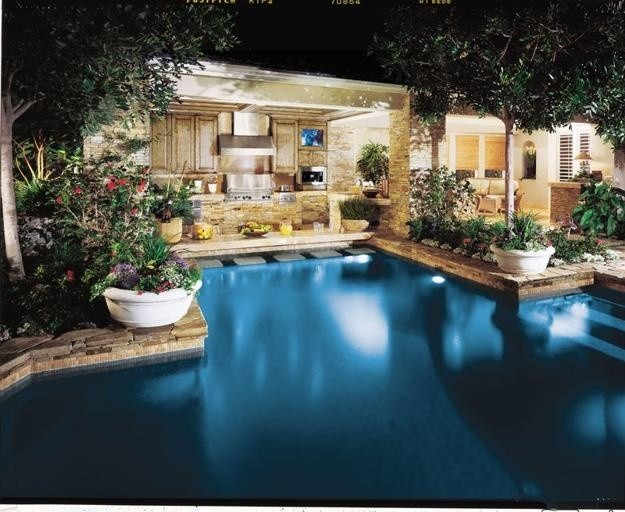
top-left (574, 152), bottom-right (593, 170)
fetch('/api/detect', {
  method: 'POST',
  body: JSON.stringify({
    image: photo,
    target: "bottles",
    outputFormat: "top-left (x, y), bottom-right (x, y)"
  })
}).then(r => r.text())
top-left (279, 218), bottom-right (292, 236)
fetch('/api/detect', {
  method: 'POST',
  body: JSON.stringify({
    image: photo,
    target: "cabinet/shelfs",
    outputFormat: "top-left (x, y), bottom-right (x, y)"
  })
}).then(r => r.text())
top-left (151, 113), bottom-right (217, 172)
top-left (272, 118), bottom-right (298, 174)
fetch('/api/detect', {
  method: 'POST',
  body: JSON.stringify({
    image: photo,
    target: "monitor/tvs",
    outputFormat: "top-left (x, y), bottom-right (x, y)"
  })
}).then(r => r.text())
top-left (300, 127), bottom-right (325, 148)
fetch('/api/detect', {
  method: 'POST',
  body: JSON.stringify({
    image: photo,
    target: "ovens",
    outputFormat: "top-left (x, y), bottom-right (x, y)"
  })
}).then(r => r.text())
top-left (297, 165), bottom-right (327, 190)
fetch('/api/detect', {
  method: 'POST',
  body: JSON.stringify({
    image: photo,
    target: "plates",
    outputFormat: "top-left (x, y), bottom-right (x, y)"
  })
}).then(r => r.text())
top-left (241, 231), bottom-right (266, 237)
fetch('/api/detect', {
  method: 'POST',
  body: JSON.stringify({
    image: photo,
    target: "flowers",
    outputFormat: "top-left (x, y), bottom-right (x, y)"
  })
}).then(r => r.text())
top-left (206, 173), bottom-right (218, 184)
top-left (38, 160), bottom-right (203, 303)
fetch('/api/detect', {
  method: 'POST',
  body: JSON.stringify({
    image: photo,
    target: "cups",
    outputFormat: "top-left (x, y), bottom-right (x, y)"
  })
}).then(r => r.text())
top-left (208, 184), bottom-right (217, 193)
top-left (194, 180), bottom-right (202, 193)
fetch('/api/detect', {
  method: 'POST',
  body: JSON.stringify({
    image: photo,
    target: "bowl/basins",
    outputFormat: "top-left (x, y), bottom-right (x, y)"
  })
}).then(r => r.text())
top-left (363, 190), bottom-right (378, 198)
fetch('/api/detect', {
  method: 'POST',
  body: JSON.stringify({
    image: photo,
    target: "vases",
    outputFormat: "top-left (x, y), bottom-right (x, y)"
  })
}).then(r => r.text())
top-left (101, 280), bottom-right (203, 329)
top-left (208, 183), bottom-right (217, 195)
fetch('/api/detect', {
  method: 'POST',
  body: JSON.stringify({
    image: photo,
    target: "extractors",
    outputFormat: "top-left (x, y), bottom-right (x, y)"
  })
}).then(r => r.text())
top-left (218, 112), bottom-right (275, 156)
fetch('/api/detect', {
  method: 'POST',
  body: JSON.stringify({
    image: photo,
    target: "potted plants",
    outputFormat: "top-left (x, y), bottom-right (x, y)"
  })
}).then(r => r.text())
top-left (489, 206), bottom-right (556, 277)
top-left (338, 194), bottom-right (376, 232)
